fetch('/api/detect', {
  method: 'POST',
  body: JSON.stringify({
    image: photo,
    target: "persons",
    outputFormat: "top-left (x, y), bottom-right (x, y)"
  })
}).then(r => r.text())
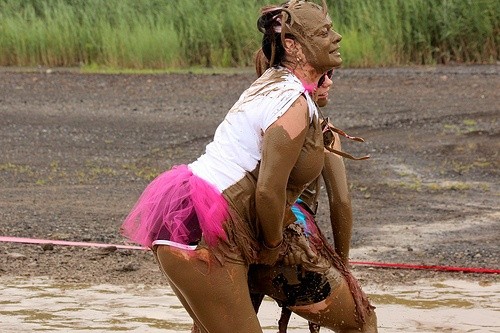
top-left (121, 0), bottom-right (343, 332)
top-left (248, 45), bottom-right (378, 333)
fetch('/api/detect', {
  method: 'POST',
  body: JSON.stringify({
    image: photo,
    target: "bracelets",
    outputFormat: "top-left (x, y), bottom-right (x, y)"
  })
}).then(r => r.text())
top-left (263, 239), bottom-right (284, 251)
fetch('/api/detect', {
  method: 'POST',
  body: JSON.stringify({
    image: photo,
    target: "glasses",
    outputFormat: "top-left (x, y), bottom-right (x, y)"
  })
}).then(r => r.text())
top-left (317, 68), bottom-right (334, 88)
top-left (321, 116), bottom-right (370, 160)
